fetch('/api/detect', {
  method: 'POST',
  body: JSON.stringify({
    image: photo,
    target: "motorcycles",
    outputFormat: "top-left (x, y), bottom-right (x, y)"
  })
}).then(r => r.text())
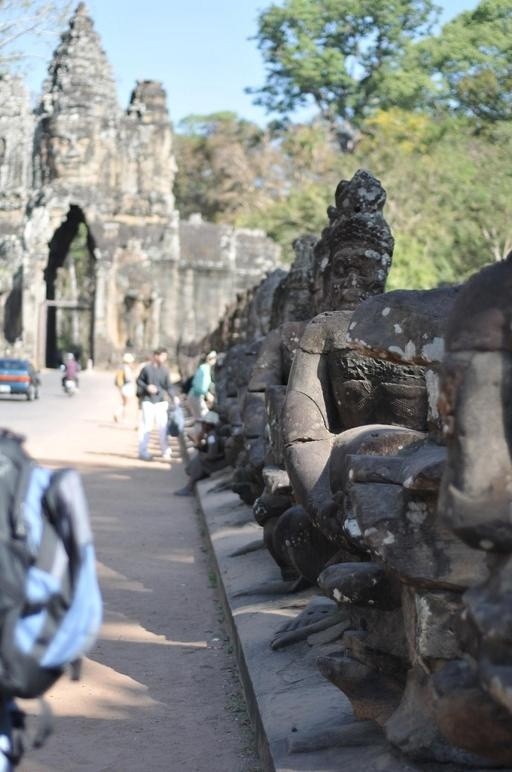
top-left (60, 363), bottom-right (82, 397)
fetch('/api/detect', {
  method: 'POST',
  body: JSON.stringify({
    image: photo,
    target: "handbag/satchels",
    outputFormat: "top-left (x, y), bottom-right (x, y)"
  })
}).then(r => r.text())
top-left (169, 406), bottom-right (184, 436)
top-left (116, 369), bottom-right (125, 386)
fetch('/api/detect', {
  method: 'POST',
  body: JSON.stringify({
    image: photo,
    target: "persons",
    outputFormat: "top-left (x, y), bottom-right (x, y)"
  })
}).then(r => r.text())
top-left (60, 353), bottom-right (82, 392)
top-left (169, 350), bottom-right (222, 450)
top-left (234, 170), bottom-right (512, 770)
top-left (32, 2), bottom-right (119, 178)
top-left (176, 289), bottom-right (256, 495)
top-left (113, 352), bottom-right (134, 423)
top-left (136, 349), bottom-right (179, 462)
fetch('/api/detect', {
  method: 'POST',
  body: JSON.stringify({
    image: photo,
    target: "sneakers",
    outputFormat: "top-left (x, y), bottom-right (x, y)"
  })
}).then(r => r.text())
top-left (175, 487), bottom-right (194, 496)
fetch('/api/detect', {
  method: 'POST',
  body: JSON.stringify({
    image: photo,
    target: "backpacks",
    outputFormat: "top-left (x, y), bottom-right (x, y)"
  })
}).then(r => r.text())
top-left (0, 428), bottom-right (102, 699)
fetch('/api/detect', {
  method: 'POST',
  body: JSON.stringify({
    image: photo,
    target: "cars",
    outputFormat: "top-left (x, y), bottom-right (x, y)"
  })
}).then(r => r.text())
top-left (0, 359), bottom-right (41, 401)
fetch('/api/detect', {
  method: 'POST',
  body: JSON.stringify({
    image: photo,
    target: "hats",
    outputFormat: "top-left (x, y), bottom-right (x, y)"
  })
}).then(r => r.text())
top-left (196, 411), bottom-right (221, 424)
top-left (123, 353), bottom-right (135, 362)
